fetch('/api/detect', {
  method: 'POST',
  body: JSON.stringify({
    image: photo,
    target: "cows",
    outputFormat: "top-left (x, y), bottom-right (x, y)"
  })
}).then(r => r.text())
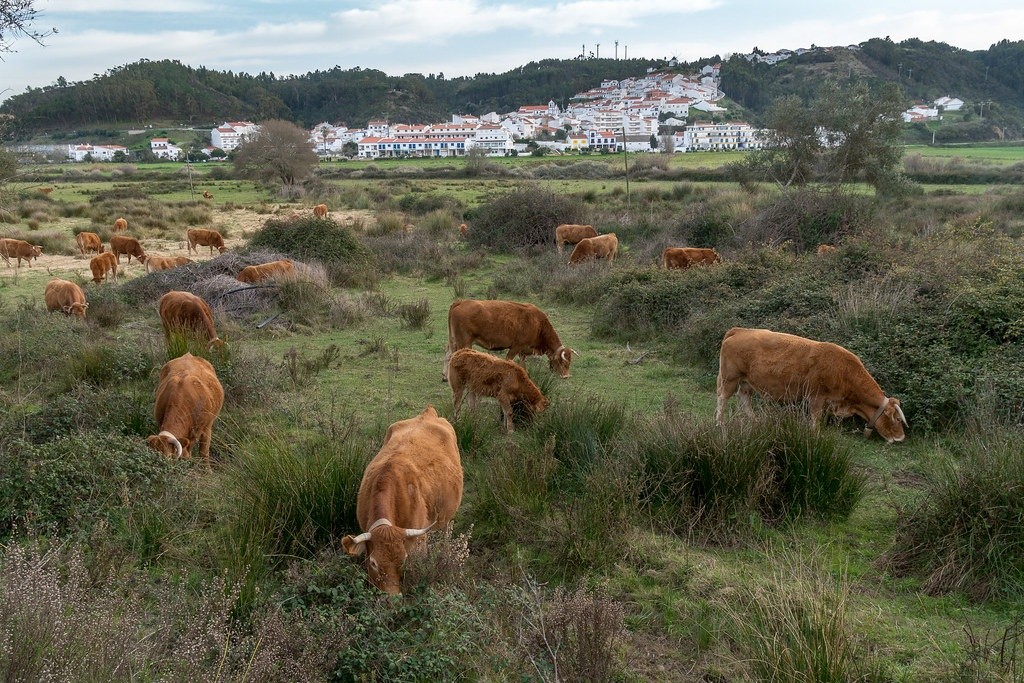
top-left (714, 326), bottom-right (910, 449)
top-left (556, 224), bottom-right (617, 266)
top-left (45, 277), bottom-right (91, 323)
top-left (314, 204), bottom-right (327, 221)
top-left (158, 291), bottom-right (232, 358)
top-left (142, 351), bottom-right (225, 476)
top-left (340, 404), bottom-right (463, 608)
top-left (237, 259), bottom-right (297, 287)
top-left (202, 191), bottom-right (213, 200)
top-left (660, 248), bottom-right (723, 271)
top-left (0, 238), bottom-right (44, 269)
top-left (440, 299), bottom-right (580, 437)
top-left (75, 217), bottom-right (230, 286)
